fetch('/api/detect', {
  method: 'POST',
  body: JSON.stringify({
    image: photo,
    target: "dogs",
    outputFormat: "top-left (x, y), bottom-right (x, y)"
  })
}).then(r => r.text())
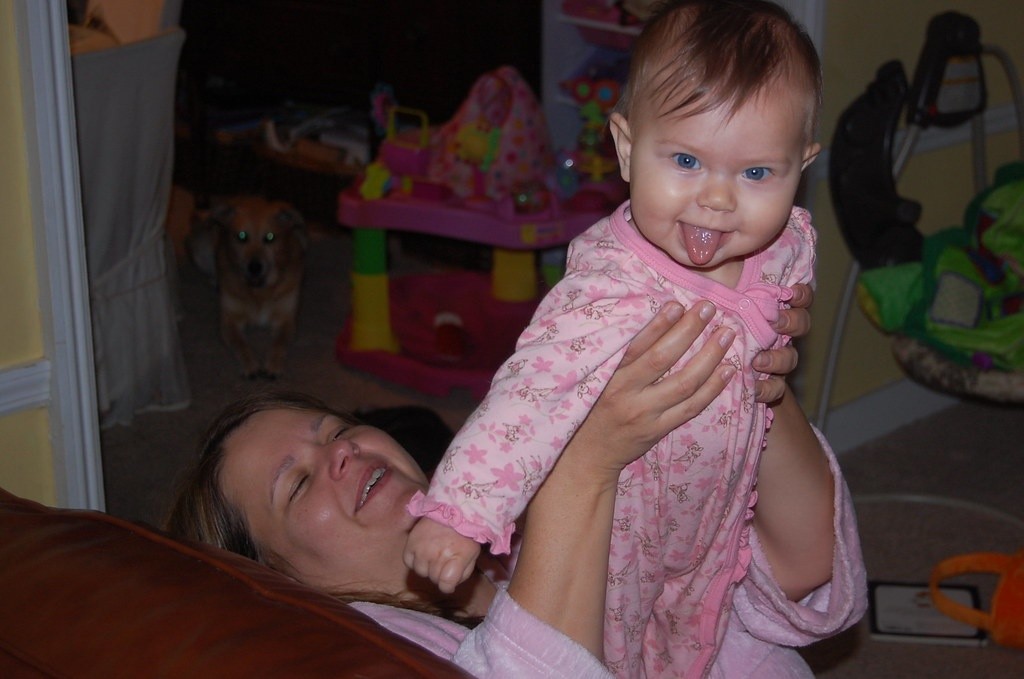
top-left (190, 193), bottom-right (319, 384)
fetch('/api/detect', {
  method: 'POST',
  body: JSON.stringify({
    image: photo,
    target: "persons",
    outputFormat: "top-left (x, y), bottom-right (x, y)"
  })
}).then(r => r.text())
top-left (170, 284), bottom-right (870, 678)
top-left (404, 0), bottom-right (821, 679)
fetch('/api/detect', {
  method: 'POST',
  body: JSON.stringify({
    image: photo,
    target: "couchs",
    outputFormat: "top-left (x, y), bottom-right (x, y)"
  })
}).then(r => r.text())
top-left (0, 487), bottom-right (478, 679)
